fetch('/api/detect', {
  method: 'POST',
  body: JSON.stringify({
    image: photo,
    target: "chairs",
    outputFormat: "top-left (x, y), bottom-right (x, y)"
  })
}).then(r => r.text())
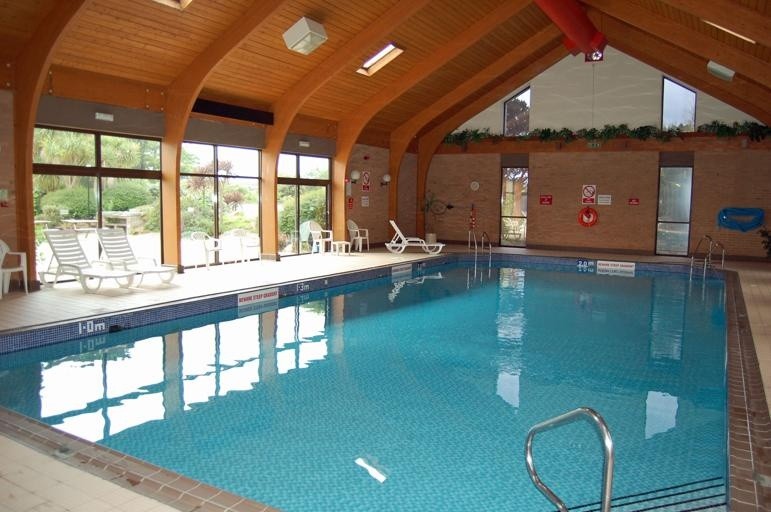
top-left (308, 218), bottom-right (446, 255)
top-left (190, 229), bottom-right (262, 270)
top-left (1, 226), bottom-right (178, 300)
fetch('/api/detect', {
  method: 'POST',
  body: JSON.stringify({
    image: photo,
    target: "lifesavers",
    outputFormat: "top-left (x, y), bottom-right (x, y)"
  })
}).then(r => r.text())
top-left (576, 207), bottom-right (598, 228)
